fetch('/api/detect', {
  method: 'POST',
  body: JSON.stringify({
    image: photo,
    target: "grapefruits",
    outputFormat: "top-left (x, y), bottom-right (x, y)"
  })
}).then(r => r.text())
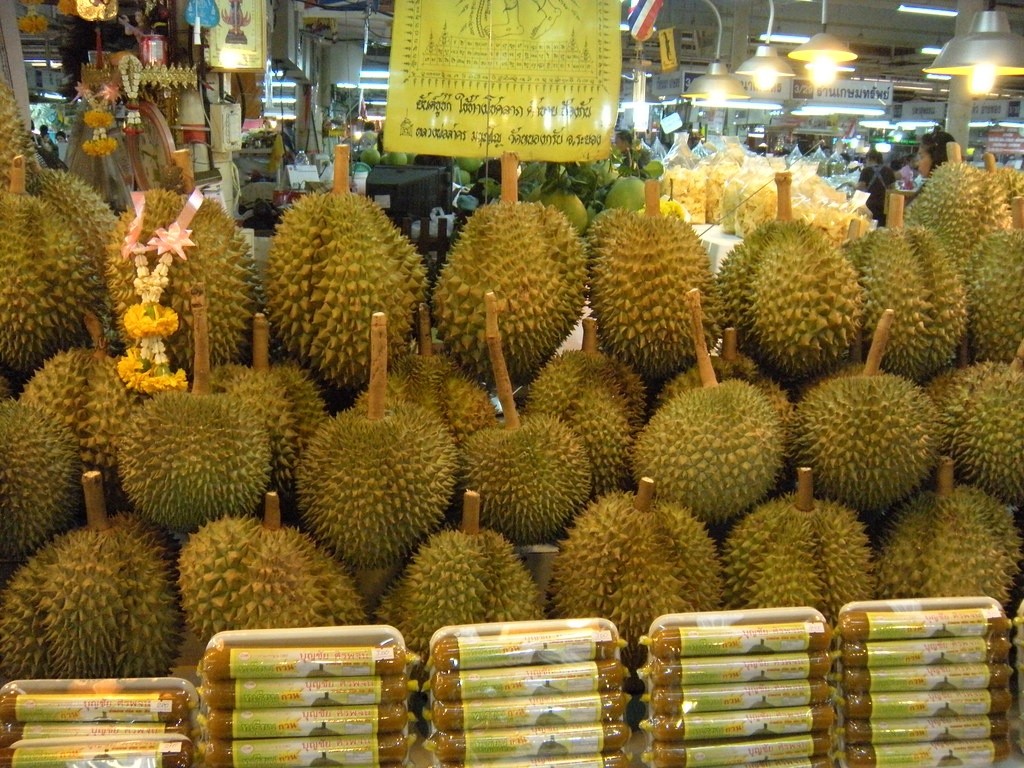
top-left (357, 130), bottom-right (644, 235)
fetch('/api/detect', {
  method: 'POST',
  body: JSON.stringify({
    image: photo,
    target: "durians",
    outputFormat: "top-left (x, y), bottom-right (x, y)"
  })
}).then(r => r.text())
top-left (0, 61), bottom-right (1024, 680)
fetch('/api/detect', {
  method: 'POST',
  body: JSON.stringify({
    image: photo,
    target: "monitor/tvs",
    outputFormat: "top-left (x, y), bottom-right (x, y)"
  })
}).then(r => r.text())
top-left (364, 164), bottom-right (452, 216)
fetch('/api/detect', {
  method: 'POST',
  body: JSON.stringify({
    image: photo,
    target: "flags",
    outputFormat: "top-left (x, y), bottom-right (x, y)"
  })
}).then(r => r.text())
top-left (627, 0), bottom-right (662, 40)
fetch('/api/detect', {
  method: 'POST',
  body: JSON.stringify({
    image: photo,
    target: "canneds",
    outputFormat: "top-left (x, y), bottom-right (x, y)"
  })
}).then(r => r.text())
top-left (139, 34), bottom-right (167, 68)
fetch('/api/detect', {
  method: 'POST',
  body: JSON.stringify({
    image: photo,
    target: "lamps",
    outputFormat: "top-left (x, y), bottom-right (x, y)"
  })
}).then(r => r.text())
top-left (680, 0), bottom-right (1024, 103)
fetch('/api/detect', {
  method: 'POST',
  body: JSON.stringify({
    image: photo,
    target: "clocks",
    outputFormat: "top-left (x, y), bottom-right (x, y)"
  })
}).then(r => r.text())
top-left (128, 100), bottom-right (175, 191)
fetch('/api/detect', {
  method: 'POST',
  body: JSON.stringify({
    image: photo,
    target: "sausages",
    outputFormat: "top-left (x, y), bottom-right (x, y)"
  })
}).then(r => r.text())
top-left (0, 596), bottom-right (1017, 768)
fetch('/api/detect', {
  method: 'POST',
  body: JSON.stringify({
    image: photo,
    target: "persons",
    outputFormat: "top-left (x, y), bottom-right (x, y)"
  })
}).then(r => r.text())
top-left (918, 131), bottom-right (956, 178)
top-left (359, 122), bottom-right (380, 148)
top-left (855, 151), bottom-right (896, 227)
top-left (615, 132), bottom-right (632, 148)
top-left (30, 120), bottom-right (68, 158)
